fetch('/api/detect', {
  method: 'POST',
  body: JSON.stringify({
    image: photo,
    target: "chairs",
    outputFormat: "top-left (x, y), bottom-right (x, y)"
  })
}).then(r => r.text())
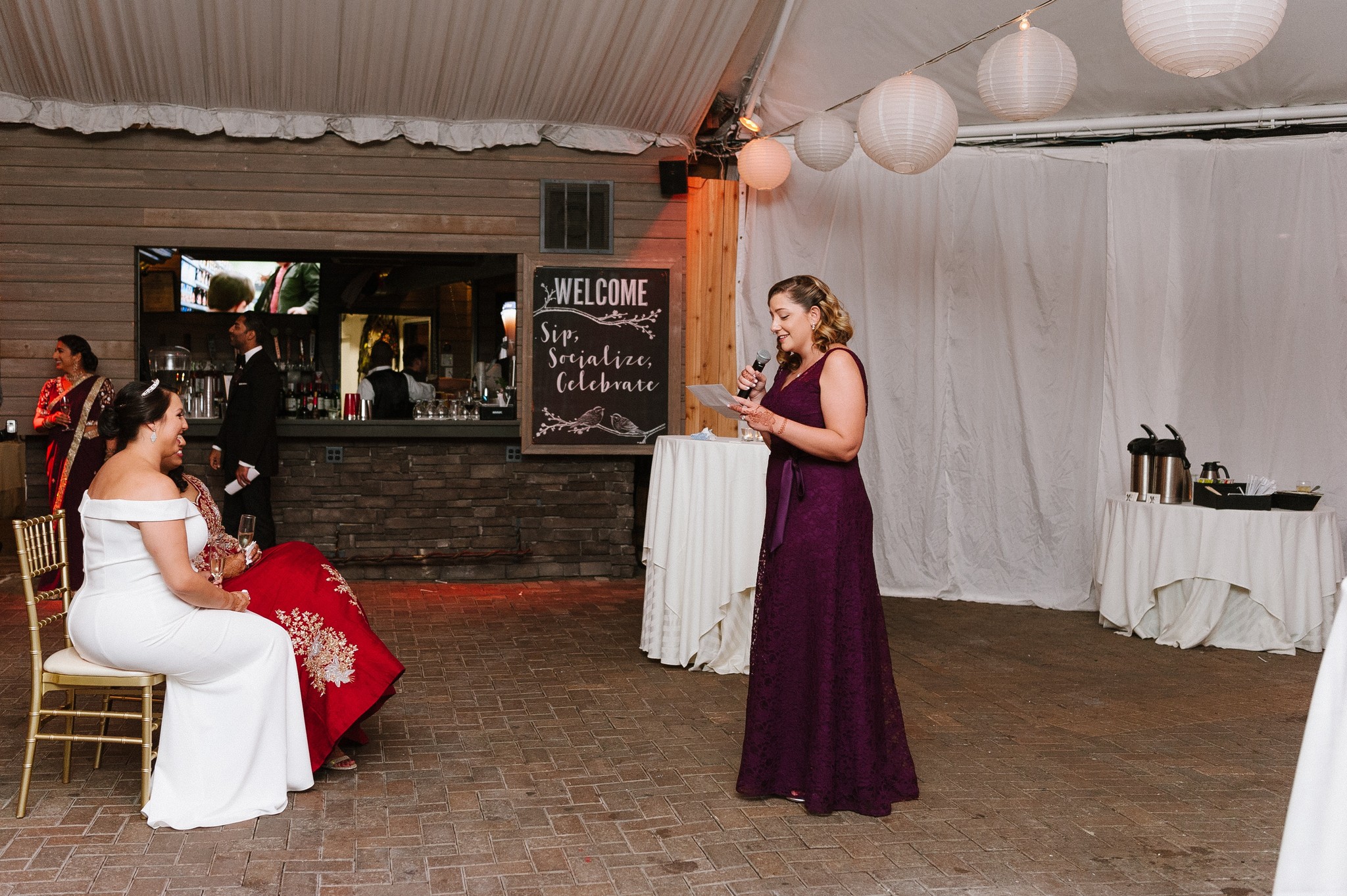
top-left (8, 509), bottom-right (166, 815)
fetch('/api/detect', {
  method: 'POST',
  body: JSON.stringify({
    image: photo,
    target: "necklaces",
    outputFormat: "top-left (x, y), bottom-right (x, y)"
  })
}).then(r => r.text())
top-left (796, 348), bottom-right (819, 378)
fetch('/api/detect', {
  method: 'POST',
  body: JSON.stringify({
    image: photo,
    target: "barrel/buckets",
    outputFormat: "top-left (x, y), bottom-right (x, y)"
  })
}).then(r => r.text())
top-left (1127, 422), bottom-right (1193, 504)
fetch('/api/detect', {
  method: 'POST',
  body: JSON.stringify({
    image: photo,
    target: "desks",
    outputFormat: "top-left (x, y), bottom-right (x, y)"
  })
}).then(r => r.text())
top-left (640, 429), bottom-right (771, 680)
top-left (1100, 489), bottom-right (1343, 653)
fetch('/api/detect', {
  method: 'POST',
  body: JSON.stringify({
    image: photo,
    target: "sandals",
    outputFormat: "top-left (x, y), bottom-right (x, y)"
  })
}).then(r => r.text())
top-left (321, 753), bottom-right (358, 771)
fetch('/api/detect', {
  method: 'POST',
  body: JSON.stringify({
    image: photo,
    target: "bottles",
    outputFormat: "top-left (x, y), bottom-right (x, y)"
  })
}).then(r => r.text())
top-left (281, 381), bottom-right (341, 418)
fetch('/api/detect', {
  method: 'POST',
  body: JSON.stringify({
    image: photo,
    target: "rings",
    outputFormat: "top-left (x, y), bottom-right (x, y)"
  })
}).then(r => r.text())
top-left (742, 415), bottom-right (745, 420)
top-left (257, 550), bottom-right (262, 553)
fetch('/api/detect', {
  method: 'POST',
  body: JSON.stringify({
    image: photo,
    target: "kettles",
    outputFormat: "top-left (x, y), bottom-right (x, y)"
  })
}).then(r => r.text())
top-left (1199, 461), bottom-right (1230, 479)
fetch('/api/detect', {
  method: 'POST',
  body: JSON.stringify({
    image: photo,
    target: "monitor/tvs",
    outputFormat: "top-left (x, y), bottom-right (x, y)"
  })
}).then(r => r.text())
top-left (177, 251), bottom-right (329, 322)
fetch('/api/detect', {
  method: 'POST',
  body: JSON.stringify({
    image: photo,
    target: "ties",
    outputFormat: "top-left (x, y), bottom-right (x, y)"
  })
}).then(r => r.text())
top-left (232, 355), bottom-right (245, 387)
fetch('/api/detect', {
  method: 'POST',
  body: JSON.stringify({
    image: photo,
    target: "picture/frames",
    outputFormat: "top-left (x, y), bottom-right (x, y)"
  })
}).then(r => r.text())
top-left (522, 249), bottom-right (685, 457)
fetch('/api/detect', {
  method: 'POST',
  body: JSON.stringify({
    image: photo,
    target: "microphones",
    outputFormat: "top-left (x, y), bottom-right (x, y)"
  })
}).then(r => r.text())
top-left (737, 348), bottom-right (772, 401)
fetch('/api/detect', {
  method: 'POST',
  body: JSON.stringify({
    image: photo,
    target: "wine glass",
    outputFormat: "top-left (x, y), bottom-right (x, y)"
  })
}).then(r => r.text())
top-left (208, 540), bottom-right (226, 581)
top-left (59, 394), bottom-right (74, 432)
top-left (238, 514), bottom-right (256, 553)
top-left (412, 392), bottom-right (480, 420)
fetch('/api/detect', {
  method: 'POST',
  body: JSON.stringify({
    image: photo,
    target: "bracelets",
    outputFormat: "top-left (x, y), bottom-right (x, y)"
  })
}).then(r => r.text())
top-left (105, 448), bottom-right (117, 453)
top-left (42, 414), bottom-right (56, 430)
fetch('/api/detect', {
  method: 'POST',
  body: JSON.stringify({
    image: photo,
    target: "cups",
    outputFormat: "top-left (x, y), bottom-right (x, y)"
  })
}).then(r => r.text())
top-left (741, 427), bottom-right (753, 442)
top-left (189, 375), bottom-right (216, 418)
top-left (756, 431), bottom-right (764, 441)
top-left (1296, 480), bottom-right (1311, 492)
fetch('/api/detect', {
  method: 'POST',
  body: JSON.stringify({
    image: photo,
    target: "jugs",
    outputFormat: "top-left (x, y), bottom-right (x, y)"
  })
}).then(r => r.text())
top-left (343, 393), bottom-right (360, 421)
top-left (359, 399), bottom-right (374, 420)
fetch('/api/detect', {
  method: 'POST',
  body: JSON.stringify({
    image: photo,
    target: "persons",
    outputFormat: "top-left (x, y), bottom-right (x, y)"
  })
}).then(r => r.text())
top-left (725, 275), bottom-right (920, 819)
top-left (207, 261), bottom-right (319, 314)
top-left (398, 343), bottom-right (429, 383)
top-left (34, 335), bottom-right (117, 602)
top-left (67, 378), bottom-right (315, 832)
top-left (210, 310), bottom-right (280, 549)
top-left (357, 340), bottom-right (436, 417)
top-left (158, 434), bottom-right (405, 770)
